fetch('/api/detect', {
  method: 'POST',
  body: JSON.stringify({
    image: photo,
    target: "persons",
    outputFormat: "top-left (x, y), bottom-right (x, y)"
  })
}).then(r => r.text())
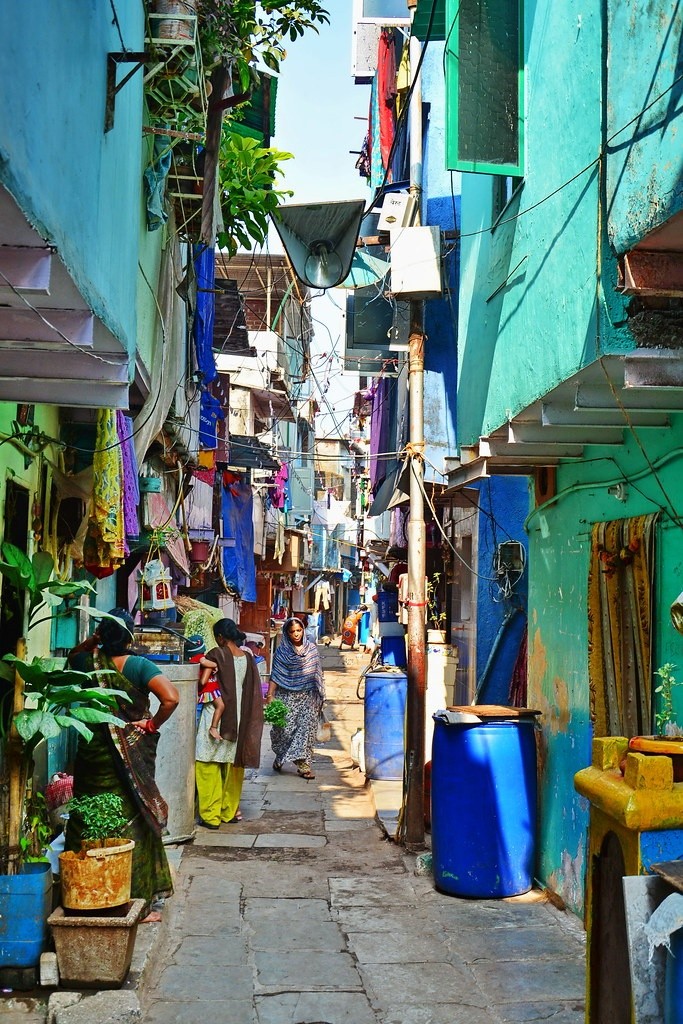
top-left (267, 617), bottom-right (326, 779)
top-left (185, 618), bottom-right (264, 830)
top-left (339, 604), bottom-right (368, 651)
top-left (63, 608), bottom-right (180, 924)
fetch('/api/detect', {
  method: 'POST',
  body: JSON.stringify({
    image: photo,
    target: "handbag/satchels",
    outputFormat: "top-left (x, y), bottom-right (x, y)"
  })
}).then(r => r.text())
top-left (315, 710), bottom-right (331, 743)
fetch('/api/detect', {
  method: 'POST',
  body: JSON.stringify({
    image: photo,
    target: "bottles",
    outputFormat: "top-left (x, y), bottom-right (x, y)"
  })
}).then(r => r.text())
top-left (351, 728), bottom-right (365, 772)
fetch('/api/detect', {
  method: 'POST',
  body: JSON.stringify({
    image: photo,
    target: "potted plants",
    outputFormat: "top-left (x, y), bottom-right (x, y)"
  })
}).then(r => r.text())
top-left (0, 542), bottom-right (132, 968)
top-left (427, 572), bottom-right (448, 644)
top-left (58, 794), bottom-right (134, 909)
top-left (185, 615), bottom-right (207, 648)
top-left (142, 0), bottom-right (331, 260)
top-left (629, 662), bottom-right (683, 783)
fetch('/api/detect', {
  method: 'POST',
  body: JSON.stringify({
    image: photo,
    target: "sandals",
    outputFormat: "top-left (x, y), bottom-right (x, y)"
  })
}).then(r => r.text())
top-left (272, 759), bottom-right (284, 770)
top-left (296, 769), bottom-right (316, 779)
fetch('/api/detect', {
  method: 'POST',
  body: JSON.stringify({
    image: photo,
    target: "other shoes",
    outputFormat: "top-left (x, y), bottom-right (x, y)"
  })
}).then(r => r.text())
top-left (338, 648), bottom-right (341, 650)
top-left (350, 648), bottom-right (355, 650)
top-left (198, 819), bottom-right (218, 829)
top-left (221, 818), bottom-right (237, 823)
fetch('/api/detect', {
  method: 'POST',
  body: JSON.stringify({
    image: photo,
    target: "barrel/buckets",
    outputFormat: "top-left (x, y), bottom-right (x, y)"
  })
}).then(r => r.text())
top-left (364, 673), bottom-right (408, 781)
top-left (430, 716), bottom-right (538, 900)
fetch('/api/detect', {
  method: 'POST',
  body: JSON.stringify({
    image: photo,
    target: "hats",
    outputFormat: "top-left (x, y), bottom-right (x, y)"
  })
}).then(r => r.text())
top-left (185, 635), bottom-right (205, 656)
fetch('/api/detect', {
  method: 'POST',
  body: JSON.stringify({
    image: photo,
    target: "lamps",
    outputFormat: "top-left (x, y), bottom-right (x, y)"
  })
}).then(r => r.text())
top-left (268, 200), bottom-right (394, 289)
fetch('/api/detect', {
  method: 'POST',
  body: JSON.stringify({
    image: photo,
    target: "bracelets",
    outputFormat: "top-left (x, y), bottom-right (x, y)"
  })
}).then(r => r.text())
top-left (267, 695), bottom-right (272, 699)
